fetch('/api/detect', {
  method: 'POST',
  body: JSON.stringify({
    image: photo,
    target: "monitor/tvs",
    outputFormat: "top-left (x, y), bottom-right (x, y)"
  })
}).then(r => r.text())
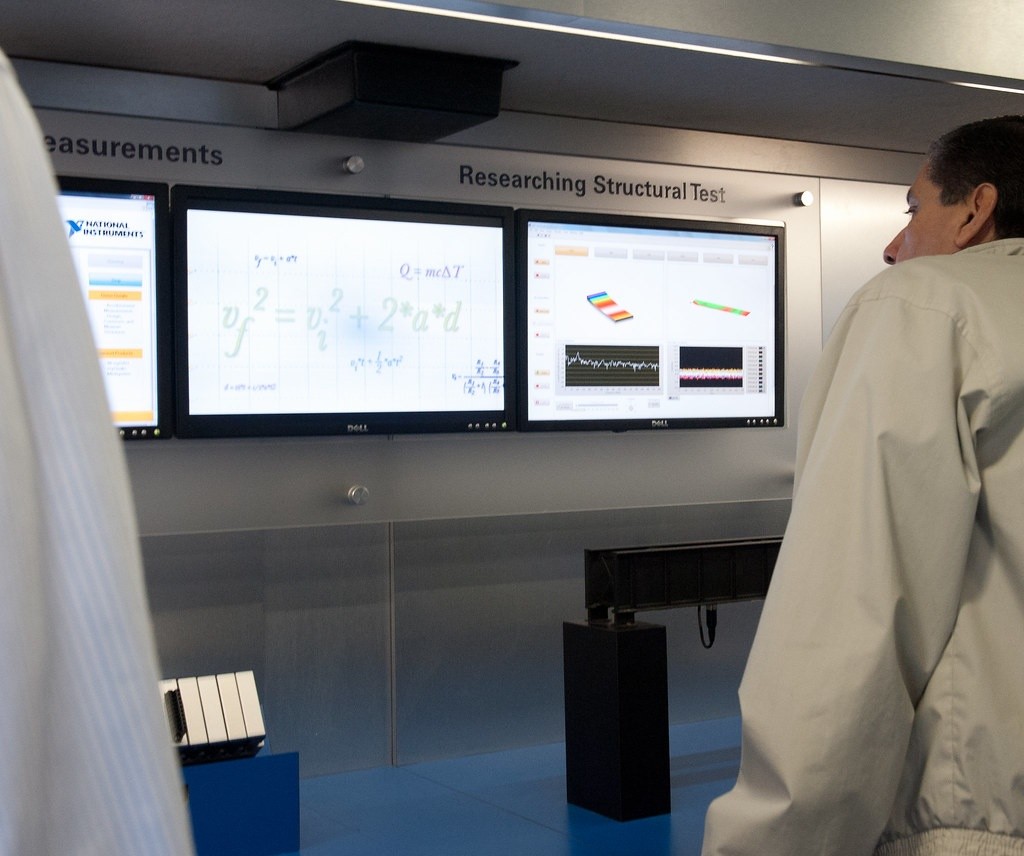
top-left (54, 174), bottom-right (785, 439)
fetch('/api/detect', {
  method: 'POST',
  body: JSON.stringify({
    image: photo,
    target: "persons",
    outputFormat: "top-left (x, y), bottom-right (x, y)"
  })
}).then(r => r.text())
top-left (701, 116), bottom-right (1024, 856)
